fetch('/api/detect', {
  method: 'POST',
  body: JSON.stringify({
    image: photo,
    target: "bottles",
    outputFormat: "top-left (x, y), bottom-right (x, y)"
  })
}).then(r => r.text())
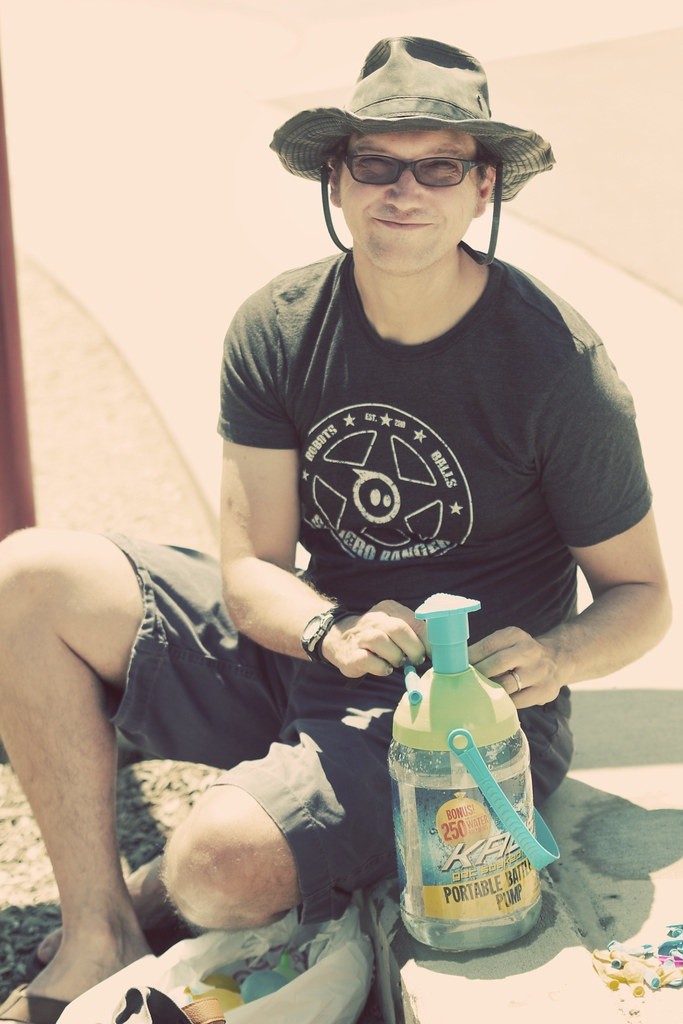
top-left (386, 593), bottom-right (542, 956)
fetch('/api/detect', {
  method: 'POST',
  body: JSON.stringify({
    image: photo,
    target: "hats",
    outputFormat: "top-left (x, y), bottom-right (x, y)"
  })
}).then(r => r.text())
top-left (268, 33), bottom-right (557, 265)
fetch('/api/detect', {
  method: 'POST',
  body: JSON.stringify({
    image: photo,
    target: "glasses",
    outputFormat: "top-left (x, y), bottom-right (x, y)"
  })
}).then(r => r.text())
top-left (340, 151), bottom-right (489, 187)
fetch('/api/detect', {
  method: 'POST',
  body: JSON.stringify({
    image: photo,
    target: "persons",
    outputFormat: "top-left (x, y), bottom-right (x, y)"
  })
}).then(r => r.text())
top-left (0, 38), bottom-right (672, 1024)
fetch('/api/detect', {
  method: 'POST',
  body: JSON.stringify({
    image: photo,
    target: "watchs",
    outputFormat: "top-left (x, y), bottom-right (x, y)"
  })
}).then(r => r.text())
top-left (301, 606), bottom-right (363, 670)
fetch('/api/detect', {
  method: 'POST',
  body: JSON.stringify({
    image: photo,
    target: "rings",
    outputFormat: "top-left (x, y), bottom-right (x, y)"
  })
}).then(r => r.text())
top-left (511, 671), bottom-right (521, 692)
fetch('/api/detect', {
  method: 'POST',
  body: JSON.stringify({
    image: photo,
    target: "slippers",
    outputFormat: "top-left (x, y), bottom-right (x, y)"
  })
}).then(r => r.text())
top-left (1, 979), bottom-right (73, 1024)
